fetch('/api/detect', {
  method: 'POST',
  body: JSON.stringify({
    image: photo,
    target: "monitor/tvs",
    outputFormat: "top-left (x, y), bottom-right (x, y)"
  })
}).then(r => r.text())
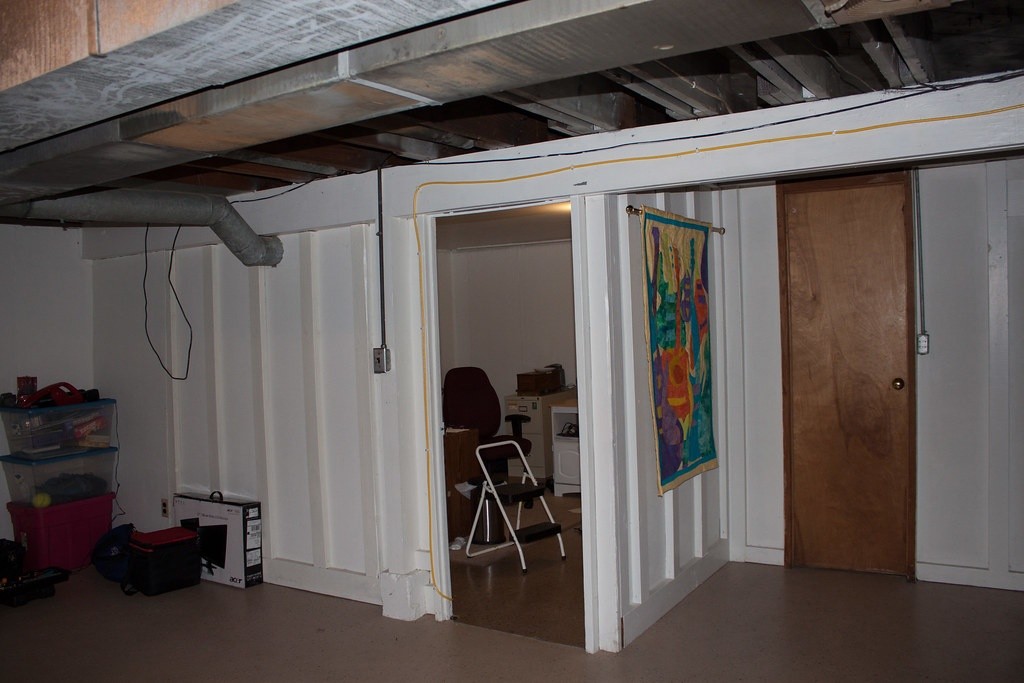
top-left (180, 517), bottom-right (200, 532)
top-left (195, 524), bottom-right (228, 575)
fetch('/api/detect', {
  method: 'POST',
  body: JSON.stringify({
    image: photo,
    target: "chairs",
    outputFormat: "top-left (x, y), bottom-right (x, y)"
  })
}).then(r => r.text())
top-left (466, 440), bottom-right (566, 573)
top-left (443, 365), bottom-right (534, 509)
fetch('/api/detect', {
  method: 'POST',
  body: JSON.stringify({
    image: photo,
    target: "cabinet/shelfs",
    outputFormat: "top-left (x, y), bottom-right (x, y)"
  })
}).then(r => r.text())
top-left (504, 387), bottom-right (581, 497)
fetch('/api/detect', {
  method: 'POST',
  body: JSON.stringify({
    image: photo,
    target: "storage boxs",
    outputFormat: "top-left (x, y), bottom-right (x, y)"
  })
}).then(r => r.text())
top-left (0, 398), bottom-right (119, 571)
top-left (173, 492), bottom-right (263, 588)
